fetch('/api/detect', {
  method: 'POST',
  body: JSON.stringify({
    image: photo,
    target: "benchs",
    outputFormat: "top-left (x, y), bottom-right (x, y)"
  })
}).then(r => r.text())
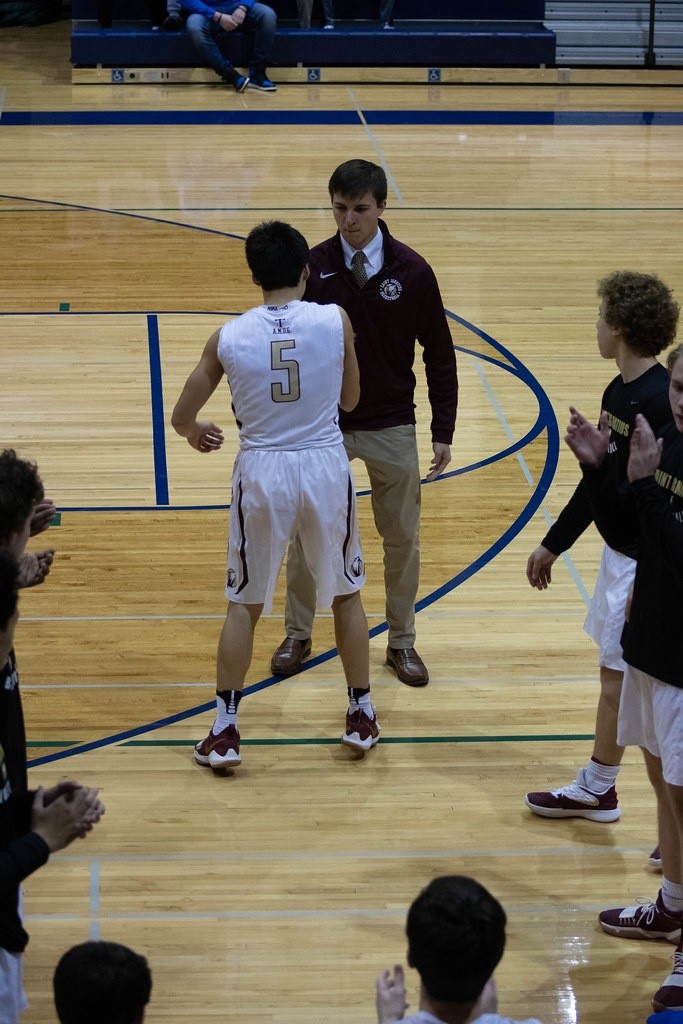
top-left (69, 0), bottom-right (557, 68)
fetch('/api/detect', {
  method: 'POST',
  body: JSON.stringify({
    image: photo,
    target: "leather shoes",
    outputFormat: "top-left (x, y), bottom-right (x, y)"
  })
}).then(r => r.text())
top-left (271, 636), bottom-right (313, 674)
top-left (387, 643), bottom-right (429, 685)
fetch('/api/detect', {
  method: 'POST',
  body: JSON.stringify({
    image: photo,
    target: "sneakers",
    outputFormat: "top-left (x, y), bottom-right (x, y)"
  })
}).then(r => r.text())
top-left (342, 707), bottom-right (382, 750)
top-left (524, 766), bottom-right (622, 821)
top-left (652, 948), bottom-right (683, 1012)
top-left (226, 74), bottom-right (251, 93)
top-left (648, 841), bottom-right (663, 867)
top-left (596, 887), bottom-right (683, 944)
top-left (247, 75), bottom-right (278, 90)
top-left (194, 717), bottom-right (241, 768)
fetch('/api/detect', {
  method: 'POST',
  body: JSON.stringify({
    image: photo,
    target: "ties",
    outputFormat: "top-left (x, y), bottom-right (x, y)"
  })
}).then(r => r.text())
top-left (351, 251), bottom-right (368, 286)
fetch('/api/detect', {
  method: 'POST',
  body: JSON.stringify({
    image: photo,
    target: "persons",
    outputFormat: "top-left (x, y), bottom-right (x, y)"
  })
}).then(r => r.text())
top-left (525, 271), bottom-right (683, 1024)
top-left (270, 158), bottom-right (458, 686)
top-left (171, 220), bottom-right (381, 768)
top-left (177, 0), bottom-right (277, 92)
top-left (96, 0), bottom-right (397, 30)
top-left (376, 875), bottom-right (543, 1024)
top-left (0, 449), bottom-right (104, 1024)
top-left (53, 940), bottom-right (153, 1024)
